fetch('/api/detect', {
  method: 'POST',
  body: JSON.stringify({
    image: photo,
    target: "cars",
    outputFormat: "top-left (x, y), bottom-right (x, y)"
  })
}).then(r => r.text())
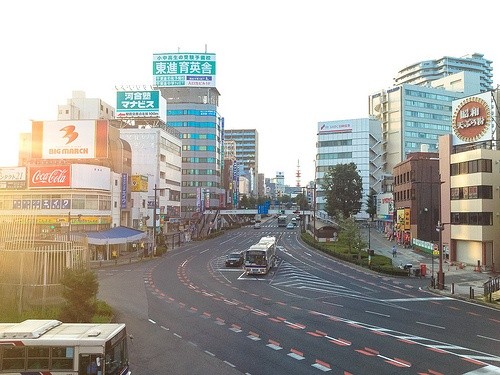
top-left (253, 223), bottom-right (262, 229)
top-left (291, 215), bottom-right (300, 226)
top-left (287, 224), bottom-right (295, 229)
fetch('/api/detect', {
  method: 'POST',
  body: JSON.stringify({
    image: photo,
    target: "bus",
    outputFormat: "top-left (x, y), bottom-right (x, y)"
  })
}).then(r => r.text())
top-left (243, 236), bottom-right (277, 275)
top-left (0, 318), bottom-right (133, 375)
top-left (278, 216), bottom-right (288, 227)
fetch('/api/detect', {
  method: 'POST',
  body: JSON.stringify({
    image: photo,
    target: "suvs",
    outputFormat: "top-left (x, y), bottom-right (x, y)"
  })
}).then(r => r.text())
top-left (225, 252), bottom-right (243, 267)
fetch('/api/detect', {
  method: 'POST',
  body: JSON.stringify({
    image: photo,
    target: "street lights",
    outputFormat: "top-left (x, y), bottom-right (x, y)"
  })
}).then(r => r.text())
top-left (436, 222), bottom-right (460, 291)
top-left (202, 189), bottom-right (216, 224)
top-left (390, 199), bottom-right (395, 240)
top-left (152, 184), bottom-right (171, 257)
top-left (307, 184), bottom-right (317, 239)
top-left (367, 221), bottom-right (371, 250)
top-left (67, 212), bottom-right (83, 241)
